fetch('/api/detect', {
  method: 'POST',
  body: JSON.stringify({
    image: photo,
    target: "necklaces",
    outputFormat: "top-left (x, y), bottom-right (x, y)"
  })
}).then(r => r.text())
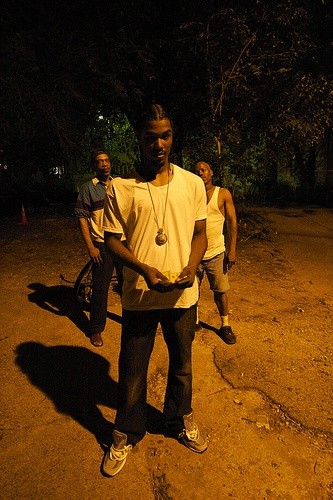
top-left (145, 164), bottom-right (170, 246)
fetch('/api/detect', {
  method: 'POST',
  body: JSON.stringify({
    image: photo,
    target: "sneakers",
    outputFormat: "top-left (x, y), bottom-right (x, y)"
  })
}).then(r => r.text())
top-left (103, 430), bottom-right (132, 477)
top-left (218, 325), bottom-right (236, 344)
top-left (195, 324), bottom-right (201, 331)
top-left (90, 332), bottom-right (103, 347)
top-left (162, 408), bottom-right (208, 454)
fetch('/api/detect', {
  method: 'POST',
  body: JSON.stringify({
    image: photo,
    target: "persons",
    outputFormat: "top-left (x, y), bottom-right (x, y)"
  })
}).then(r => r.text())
top-left (74, 150), bottom-right (127, 347)
top-left (193, 161), bottom-right (238, 345)
top-left (102, 104), bottom-right (208, 477)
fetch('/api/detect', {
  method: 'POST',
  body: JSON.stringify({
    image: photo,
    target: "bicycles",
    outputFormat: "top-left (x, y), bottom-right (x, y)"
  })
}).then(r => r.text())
top-left (60, 254), bottom-right (118, 306)
